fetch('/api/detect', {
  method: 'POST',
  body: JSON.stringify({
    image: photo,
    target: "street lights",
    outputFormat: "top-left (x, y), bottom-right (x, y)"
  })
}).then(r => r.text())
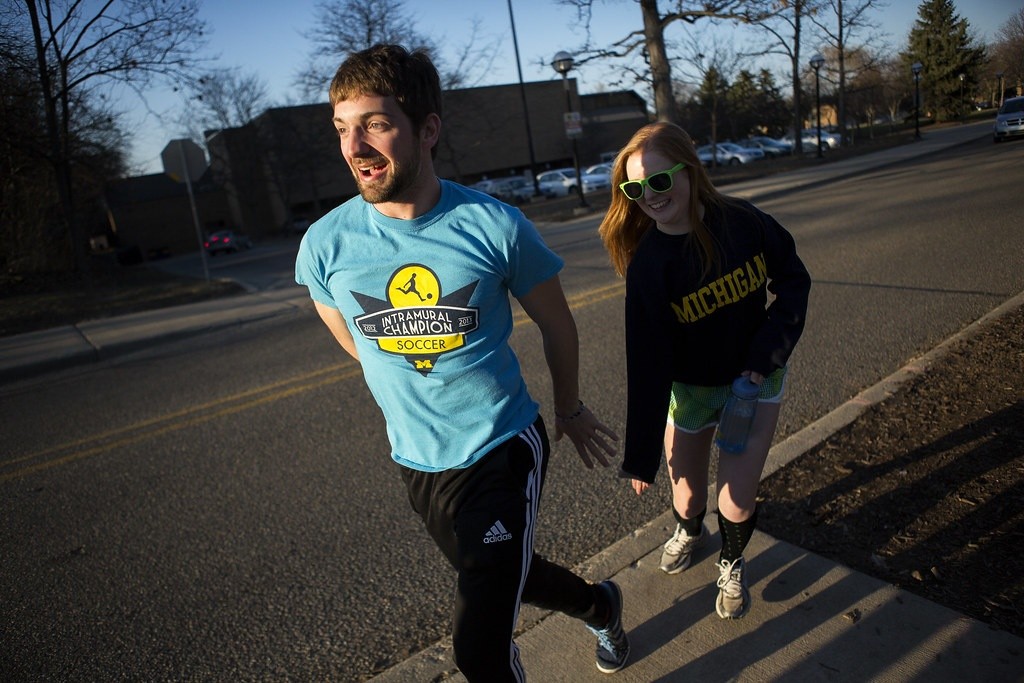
top-left (551, 51), bottom-right (591, 206)
top-left (958, 73), bottom-right (966, 123)
top-left (911, 62), bottom-right (924, 140)
top-left (810, 54), bottom-right (827, 160)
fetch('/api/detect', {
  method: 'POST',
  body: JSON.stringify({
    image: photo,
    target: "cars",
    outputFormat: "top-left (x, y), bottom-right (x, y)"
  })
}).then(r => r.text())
top-left (585, 163), bottom-right (613, 176)
top-left (205, 232), bottom-right (250, 255)
top-left (994, 96), bottom-right (1024, 142)
top-left (781, 130), bottom-right (843, 150)
top-left (737, 138), bottom-right (793, 155)
top-left (699, 143), bottom-right (763, 168)
top-left (537, 168), bottom-right (611, 198)
top-left (474, 178), bottom-right (554, 205)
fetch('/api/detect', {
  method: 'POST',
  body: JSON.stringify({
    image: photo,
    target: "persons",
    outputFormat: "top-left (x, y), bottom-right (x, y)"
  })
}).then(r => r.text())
top-left (597, 121), bottom-right (813, 619)
top-left (294, 41), bottom-right (630, 682)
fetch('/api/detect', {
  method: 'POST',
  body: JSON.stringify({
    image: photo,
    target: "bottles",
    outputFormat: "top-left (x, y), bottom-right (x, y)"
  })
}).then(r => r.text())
top-left (714, 376), bottom-right (759, 455)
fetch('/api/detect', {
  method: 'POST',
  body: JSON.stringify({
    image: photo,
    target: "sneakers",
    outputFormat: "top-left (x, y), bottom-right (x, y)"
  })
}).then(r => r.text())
top-left (658, 521), bottom-right (710, 575)
top-left (585, 578), bottom-right (631, 674)
top-left (715, 550), bottom-right (751, 619)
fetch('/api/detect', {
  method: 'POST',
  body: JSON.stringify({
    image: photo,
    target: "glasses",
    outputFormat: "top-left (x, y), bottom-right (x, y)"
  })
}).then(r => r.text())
top-left (617, 163), bottom-right (686, 201)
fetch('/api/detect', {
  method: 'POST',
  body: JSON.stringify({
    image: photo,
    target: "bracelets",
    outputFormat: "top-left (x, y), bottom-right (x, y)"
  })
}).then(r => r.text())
top-left (554, 400), bottom-right (584, 421)
